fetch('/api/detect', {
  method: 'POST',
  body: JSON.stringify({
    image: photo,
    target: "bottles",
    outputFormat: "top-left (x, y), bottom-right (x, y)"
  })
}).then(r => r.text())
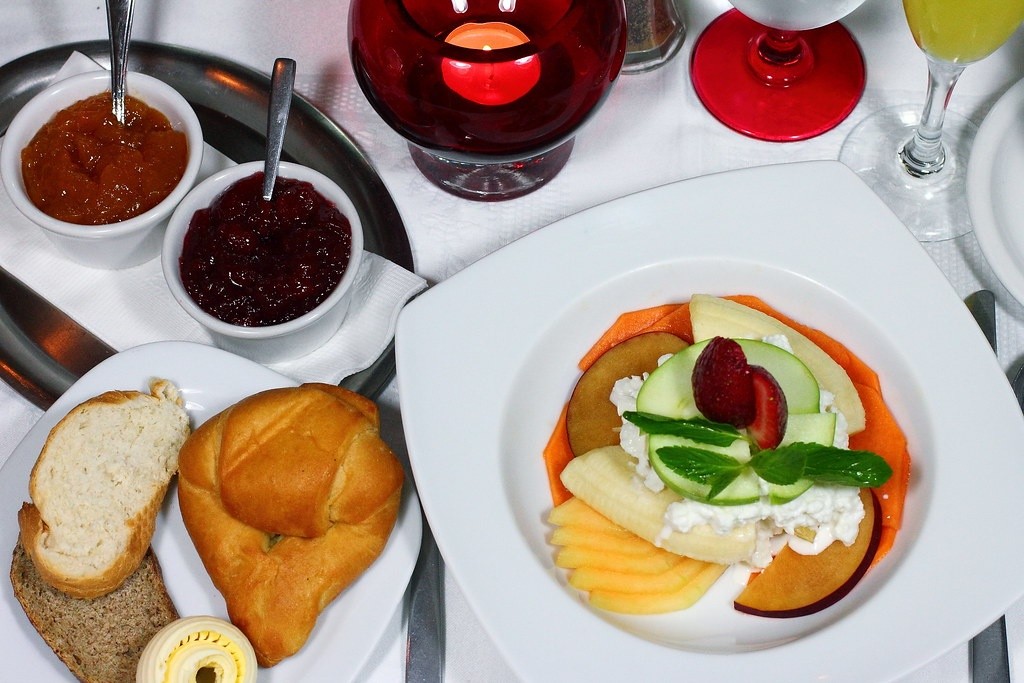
top-left (625, 0), bottom-right (688, 75)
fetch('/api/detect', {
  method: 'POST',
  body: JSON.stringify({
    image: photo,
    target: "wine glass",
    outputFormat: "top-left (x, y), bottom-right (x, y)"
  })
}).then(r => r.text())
top-left (840, 0), bottom-right (1023, 242)
top-left (347, 0), bottom-right (626, 201)
top-left (689, 0), bottom-right (868, 144)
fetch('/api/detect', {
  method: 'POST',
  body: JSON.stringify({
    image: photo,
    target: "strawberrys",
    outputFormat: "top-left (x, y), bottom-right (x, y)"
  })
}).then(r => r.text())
top-left (692, 335), bottom-right (757, 430)
top-left (744, 364), bottom-right (788, 451)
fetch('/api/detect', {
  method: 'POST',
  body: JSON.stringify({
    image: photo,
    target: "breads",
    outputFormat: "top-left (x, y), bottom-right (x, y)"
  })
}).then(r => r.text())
top-left (10, 380), bottom-right (403, 683)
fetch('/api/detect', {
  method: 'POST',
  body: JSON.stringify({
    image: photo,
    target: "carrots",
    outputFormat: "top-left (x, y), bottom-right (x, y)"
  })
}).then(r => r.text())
top-left (543, 296), bottom-right (910, 570)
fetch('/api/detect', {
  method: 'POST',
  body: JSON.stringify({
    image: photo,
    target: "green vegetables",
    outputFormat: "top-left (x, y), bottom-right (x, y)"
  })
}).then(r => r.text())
top-left (620, 409), bottom-right (762, 457)
top-left (653, 442), bottom-right (893, 488)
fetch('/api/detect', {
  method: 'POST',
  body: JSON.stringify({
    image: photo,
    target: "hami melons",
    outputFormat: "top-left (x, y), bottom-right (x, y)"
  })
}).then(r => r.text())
top-left (546, 497), bottom-right (732, 613)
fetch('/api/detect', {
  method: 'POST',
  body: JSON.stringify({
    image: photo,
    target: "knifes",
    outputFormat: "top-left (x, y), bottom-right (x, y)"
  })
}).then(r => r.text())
top-left (965, 288), bottom-right (1012, 682)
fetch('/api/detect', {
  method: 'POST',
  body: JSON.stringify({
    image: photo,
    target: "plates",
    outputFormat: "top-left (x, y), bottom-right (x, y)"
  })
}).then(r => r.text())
top-left (395, 161), bottom-right (1023, 683)
top-left (1, 341), bottom-right (422, 683)
top-left (0, 39), bottom-right (416, 405)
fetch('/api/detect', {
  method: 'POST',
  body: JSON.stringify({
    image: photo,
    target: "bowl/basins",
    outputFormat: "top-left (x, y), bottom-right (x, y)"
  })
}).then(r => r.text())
top-left (1, 71), bottom-right (203, 267)
top-left (161, 161), bottom-right (364, 338)
